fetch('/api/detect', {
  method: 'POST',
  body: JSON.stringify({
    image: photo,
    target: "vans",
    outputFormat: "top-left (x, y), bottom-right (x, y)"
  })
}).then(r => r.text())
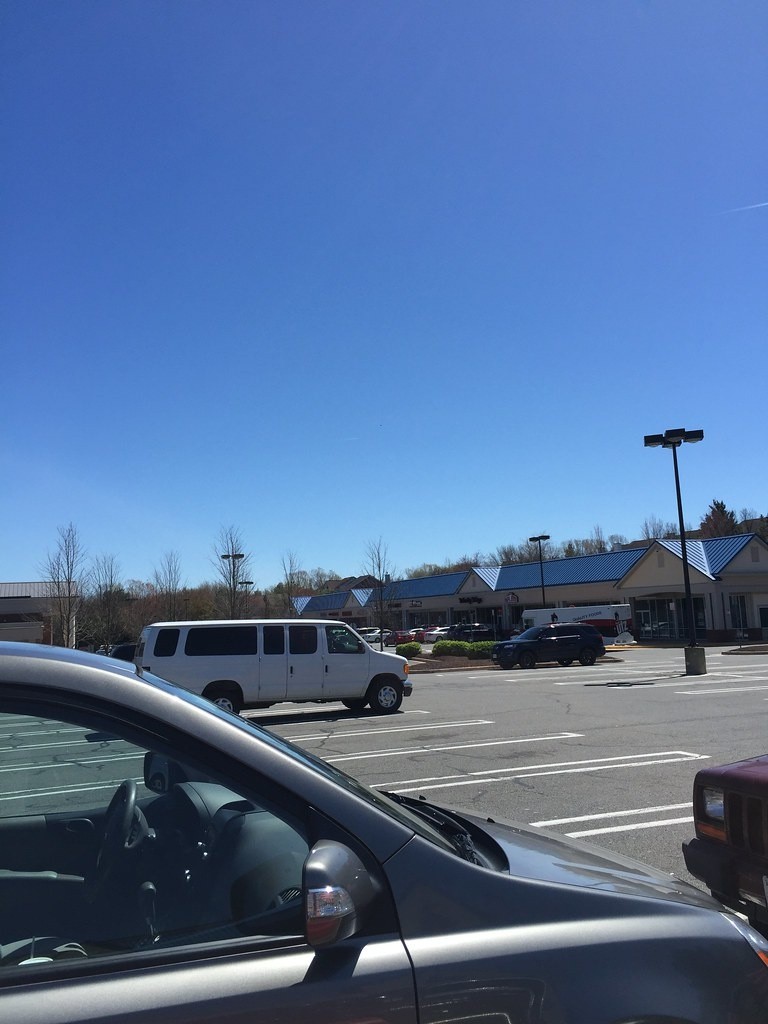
top-left (331, 627), bottom-right (379, 639)
top-left (136, 619), bottom-right (412, 716)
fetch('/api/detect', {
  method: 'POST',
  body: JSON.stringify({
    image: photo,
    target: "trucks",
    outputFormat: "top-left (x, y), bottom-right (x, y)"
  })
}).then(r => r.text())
top-left (520, 603), bottom-right (632, 637)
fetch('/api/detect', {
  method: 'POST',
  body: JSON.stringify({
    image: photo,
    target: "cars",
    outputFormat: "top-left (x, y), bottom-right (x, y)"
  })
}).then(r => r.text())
top-left (0, 641), bottom-right (768, 1024)
top-left (406, 627), bottom-right (441, 643)
top-left (363, 629), bottom-right (392, 643)
top-left (384, 630), bottom-right (412, 646)
top-left (683, 751), bottom-right (768, 940)
top-left (424, 626), bottom-right (450, 643)
top-left (95, 643), bottom-right (135, 663)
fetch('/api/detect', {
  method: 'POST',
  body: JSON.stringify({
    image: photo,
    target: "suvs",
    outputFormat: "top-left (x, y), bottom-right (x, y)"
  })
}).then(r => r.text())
top-left (447, 623), bottom-right (503, 642)
top-left (490, 622), bottom-right (606, 670)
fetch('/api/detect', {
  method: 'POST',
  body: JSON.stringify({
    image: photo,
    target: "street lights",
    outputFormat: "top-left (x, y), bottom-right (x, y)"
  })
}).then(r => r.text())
top-left (222, 554), bottom-right (243, 616)
top-left (183, 599), bottom-right (190, 619)
top-left (644, 429), bottom-right (709, 675)
top-left (530, 535), bottom-right (552, 609)
top-left (239, 582), bottom-right (253, 616)
